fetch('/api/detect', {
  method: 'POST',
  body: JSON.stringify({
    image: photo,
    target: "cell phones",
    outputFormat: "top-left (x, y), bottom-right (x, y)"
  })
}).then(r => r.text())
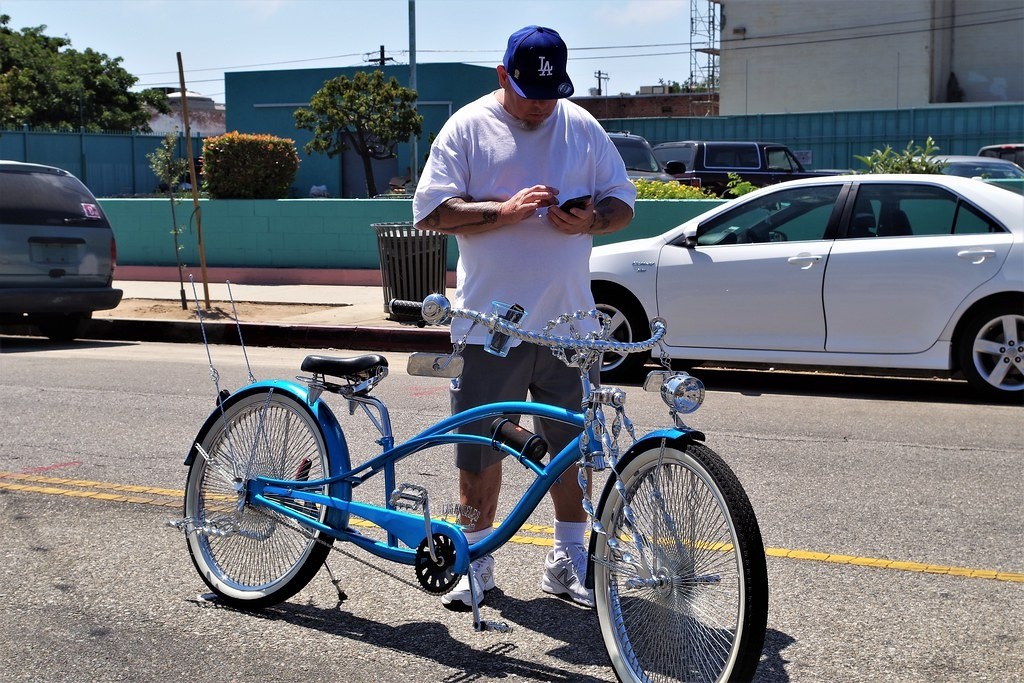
top-left (558, 195), bottom-right (592, 215)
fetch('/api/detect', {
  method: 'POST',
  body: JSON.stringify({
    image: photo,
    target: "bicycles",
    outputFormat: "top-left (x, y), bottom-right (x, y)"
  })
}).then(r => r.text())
top-left (164, 292), bottom-right (769, 683)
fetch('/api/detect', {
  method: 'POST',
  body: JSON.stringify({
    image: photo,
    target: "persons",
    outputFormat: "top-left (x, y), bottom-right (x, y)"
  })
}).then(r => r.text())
top-left (412, 25), bottom-right (637, 610)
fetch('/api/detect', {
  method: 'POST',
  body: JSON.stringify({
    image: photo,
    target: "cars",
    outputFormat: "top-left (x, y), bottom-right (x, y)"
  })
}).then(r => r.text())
top-left (585, 175), bottom-right (1024, 407)
top-left (872, 154), bottom-right (1024, 193)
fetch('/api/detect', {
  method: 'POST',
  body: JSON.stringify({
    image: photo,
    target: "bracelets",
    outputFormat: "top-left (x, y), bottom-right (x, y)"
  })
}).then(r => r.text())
top-left (589, 210), bottom-right (598, 230)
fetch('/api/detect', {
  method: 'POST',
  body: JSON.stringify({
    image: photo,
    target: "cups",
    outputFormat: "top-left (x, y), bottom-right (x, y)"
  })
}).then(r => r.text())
top-left (484, 301), bottom-right (528, 357)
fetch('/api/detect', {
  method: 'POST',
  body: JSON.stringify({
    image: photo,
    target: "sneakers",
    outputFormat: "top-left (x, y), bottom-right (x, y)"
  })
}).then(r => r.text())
top-left (441, 555), bottom-right (495, 608)
top-left (542, 545), bottom-right (594, 607)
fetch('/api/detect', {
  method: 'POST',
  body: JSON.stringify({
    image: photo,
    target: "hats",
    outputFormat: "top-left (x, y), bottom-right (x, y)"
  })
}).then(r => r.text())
top-left (503, 25), bottom-right (574, 100)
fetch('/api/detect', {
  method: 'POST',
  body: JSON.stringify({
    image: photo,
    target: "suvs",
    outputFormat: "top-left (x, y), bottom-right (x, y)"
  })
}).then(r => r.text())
top-left (653, 141), bottom-right (844, 202)
top-left (0, 159), bottom-right (124, 341)
top-left (605, 131), bottom-right (678, 186)
